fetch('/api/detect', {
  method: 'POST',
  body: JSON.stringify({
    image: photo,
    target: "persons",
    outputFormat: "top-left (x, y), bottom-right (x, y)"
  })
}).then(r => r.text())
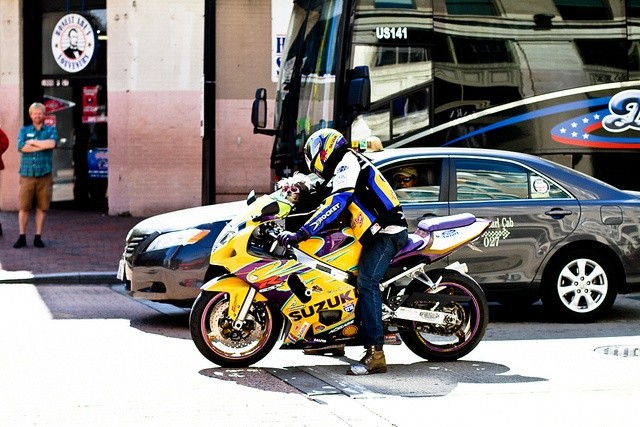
top-left (0, 128), bottom-right (9, 237)
top-left (13, 102), bottom-right (58, 248)
top-left (63, 28), bottom-right (83, 59)
top-left (277, 128), bottom-right (408, 376)
top-left (393, 167), bottom-right (418, 188)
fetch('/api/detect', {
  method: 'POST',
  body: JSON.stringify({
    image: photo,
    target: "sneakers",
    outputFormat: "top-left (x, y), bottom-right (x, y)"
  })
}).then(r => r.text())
top-left (13, 238), bottom-right (26, 248)
top-left (303, 344), bottom-right (345, 357)
top-left (34, 239), bottom-right (45, 248)
top-left (347, 345), bottom-right (387, 375)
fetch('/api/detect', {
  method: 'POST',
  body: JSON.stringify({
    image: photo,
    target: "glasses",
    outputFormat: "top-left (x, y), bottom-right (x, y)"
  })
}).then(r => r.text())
top-left (283, 184), bottom-right (300, 194)
top-left (396, 177), bottom-right (412, 183)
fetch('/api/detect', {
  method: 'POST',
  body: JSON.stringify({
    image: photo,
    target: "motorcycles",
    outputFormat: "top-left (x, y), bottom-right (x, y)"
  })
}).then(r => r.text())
top-left (190, 190), bottom-right (492, 368)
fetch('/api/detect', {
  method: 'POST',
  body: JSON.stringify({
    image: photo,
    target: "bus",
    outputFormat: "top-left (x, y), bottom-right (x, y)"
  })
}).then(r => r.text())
top-left (251, 0), bottom-right (640, 192)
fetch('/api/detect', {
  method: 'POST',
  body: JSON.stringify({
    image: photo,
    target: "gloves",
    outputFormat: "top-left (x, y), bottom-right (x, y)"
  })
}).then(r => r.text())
top-left (277, 230), bottom-right (307, 247)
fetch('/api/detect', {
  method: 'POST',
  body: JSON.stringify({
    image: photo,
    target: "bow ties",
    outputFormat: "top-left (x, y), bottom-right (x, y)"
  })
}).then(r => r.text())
top-left (71, 48), bottom-right (78, 51)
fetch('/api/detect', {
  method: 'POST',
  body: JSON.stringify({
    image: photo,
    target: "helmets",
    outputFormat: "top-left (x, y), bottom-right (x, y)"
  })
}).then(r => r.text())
top-left (303, 128), bottom-right (349, 180)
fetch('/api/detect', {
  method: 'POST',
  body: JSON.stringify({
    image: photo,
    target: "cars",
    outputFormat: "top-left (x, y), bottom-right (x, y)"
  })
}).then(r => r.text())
top-left (117, 148), bottom-right (640, 323)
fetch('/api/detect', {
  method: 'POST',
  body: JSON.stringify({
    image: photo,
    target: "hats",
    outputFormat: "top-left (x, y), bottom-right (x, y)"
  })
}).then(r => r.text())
top-left (392, 166), bottom-right (418, 179)
